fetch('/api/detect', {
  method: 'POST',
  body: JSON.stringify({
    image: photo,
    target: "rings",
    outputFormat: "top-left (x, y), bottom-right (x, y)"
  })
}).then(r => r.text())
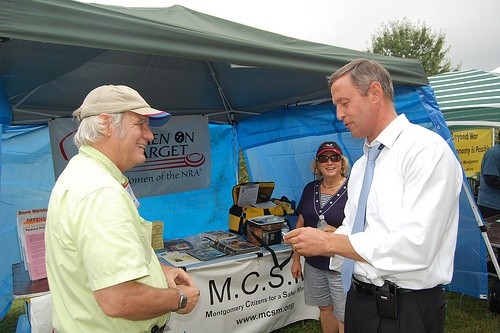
top-left (294, 243), bottom-right (296, 250)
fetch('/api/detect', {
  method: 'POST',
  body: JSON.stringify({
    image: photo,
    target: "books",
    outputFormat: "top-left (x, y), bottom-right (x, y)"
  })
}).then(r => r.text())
top-left (149, 183), bottom-right (284, 268)
top-left (16, 209), bottom-right (48, 281)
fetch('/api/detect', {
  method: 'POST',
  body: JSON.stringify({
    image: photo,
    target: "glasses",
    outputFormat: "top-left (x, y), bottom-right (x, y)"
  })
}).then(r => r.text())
top-left (316, 155), bottom-right (342, 163)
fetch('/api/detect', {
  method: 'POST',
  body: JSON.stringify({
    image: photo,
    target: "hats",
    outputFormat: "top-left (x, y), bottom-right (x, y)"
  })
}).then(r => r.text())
top-left (77, 84), bottom-right (171, 123)
top-left (316, 142), bottom-right (343, 157)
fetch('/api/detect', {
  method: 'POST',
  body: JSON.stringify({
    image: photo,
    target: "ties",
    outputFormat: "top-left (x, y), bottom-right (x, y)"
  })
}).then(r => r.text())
top-left (341, 148), bottom-right (381, 293)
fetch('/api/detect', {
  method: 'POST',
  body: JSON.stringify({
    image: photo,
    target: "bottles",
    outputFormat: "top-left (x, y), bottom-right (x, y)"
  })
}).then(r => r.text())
top-left (281, 222), bottom-right (290, 247)
top-left (317, 215), bottom-right (327, 231)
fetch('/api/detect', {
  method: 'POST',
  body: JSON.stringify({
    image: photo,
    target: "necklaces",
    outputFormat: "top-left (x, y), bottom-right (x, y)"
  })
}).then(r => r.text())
top-left (321, 183), bottom-right (340, 189)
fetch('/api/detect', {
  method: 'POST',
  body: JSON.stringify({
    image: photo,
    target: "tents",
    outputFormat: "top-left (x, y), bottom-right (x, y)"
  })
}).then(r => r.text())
top-left (424, 68), bottom-right (500, 131)
top-left (0, 0), bottom-right (500, 333)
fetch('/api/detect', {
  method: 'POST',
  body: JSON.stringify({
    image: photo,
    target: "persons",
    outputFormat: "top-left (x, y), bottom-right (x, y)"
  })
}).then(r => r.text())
top-left (43, 84), bottom-right (201, 333)
top-left (291, 141), bottom-right (351, 333)
top-left (476, 130), bottom-right (500, 219)
top-left (281, 57), bottom-right (464, 333)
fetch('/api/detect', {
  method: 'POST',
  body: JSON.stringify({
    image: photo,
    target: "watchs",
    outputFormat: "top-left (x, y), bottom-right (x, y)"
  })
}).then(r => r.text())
top-left (173, 288), bottom-right (187, 312)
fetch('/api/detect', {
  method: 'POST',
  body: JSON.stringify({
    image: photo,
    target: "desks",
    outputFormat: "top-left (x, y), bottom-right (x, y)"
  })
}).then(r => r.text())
top-left (11, 231), bottom-right (293, 327)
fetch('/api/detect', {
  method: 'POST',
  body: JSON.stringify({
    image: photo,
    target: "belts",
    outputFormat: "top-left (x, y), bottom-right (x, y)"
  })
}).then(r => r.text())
top-left (351, 278), bottom-right (441, 296)
